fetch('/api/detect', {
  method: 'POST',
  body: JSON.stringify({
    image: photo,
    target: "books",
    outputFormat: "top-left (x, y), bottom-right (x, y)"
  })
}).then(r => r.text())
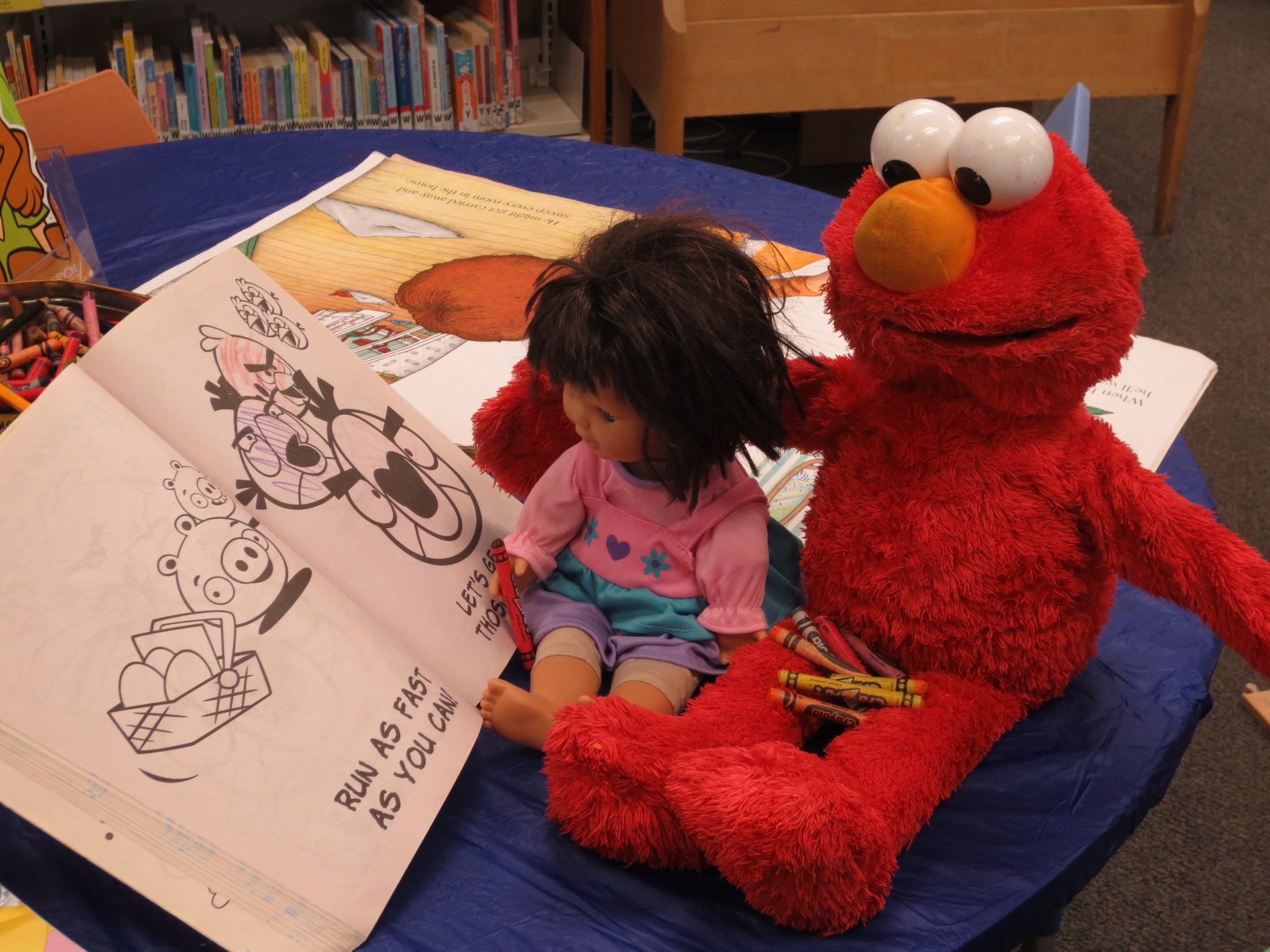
top-left (0, 7), bottom-right (521, 138)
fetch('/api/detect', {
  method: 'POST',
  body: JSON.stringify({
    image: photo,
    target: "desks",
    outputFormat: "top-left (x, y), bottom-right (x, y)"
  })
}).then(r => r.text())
top-left (2, 131), bottom-right (1221, 952)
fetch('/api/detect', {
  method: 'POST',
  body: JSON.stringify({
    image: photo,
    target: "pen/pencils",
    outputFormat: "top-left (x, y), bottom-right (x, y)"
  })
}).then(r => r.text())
top-left (0, 297), bottom-right (50, 344)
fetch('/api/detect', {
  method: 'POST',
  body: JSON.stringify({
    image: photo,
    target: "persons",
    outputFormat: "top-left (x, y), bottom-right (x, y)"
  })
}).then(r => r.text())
top-left (471, 194), bottom-right (830, 753)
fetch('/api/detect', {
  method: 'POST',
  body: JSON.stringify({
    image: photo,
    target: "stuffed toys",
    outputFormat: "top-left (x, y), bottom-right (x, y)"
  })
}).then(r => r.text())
top-left (540, 99), bottom-right (1270, 934)
